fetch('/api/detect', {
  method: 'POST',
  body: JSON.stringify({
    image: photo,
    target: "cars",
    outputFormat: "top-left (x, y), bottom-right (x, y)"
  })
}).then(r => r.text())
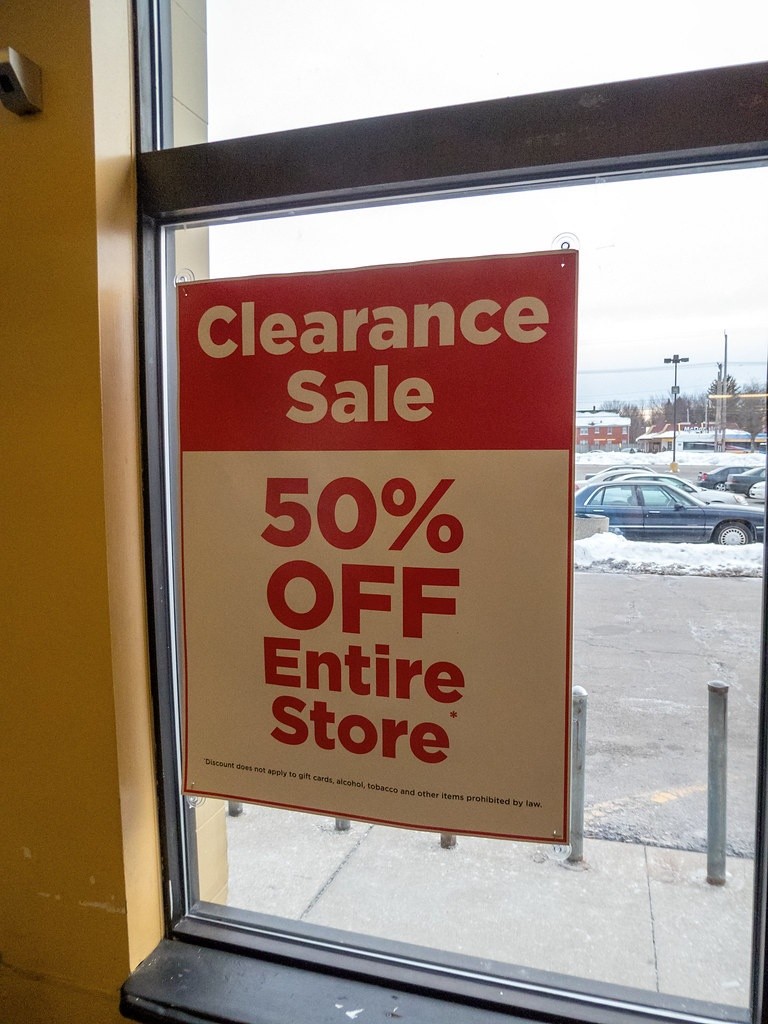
top-left (697, 464), bottom-right (754, 492)
top-left (622, 448), bottom-right (638, 453)
top-left (587, 450), bottom-right (603, 453)
top-left (725, 467), bottom-right (766, 500)
top-left (576, 465), bottom-right (749, 508)
top-left (576, 483), bottom-right (764, 546)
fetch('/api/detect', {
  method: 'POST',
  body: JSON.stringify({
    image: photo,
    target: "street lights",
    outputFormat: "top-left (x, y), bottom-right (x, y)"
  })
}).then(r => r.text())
top-left (664, 355), bottom-right (690, 472)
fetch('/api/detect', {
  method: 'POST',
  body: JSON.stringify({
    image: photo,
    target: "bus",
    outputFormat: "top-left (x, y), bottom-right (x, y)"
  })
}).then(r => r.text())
top-left (676, 433), bottom-right (766, 453)
